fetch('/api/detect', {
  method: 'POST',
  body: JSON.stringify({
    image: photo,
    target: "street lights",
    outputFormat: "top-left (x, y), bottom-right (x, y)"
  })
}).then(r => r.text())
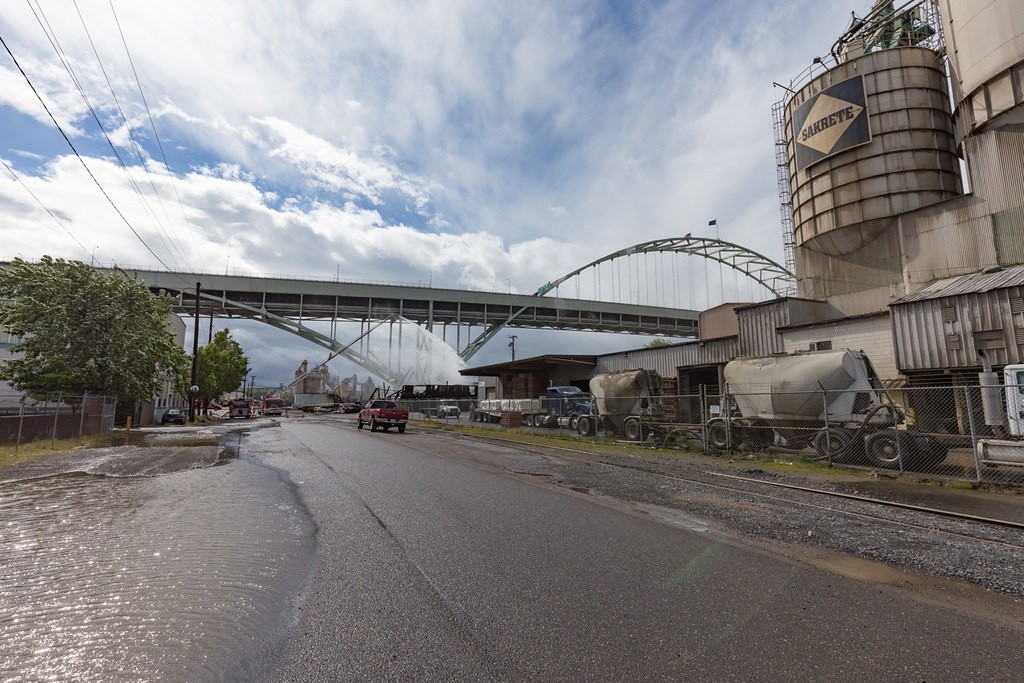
top-left (336, 264), bottom-right (340, 283)
top-left (91, 246), bottom-right (100, 268)
top-left (506, 278), bottom-right (510, 294)
top-left (429, 271), bottom-right (432, 288)
top-left (225, 256), bottom-right (231, 276)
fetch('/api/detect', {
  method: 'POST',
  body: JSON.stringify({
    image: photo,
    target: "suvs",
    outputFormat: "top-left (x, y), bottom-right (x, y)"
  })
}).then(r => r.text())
top-left (436, 398), bottom-right (462, 419)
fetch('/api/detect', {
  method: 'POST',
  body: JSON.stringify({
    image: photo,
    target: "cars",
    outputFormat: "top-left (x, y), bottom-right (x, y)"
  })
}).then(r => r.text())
top-left (195, 400), bottom-right (229, 411)
top-left (161, 408), bottom-right (187, 425)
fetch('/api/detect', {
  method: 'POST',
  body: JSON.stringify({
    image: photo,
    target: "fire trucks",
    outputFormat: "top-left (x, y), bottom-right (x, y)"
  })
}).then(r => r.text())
top-left (260, 312), bottom-right (400, 417)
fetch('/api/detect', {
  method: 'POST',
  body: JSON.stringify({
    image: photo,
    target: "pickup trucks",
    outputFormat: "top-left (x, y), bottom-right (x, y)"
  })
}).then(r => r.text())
top-left (357, 399), bottom-right (409, 434)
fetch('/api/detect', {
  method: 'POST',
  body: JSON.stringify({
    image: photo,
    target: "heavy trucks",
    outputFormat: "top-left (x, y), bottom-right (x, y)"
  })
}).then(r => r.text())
top-left (472, 385), bottom-right (596, 431)
top-left (588, 346), bottom-right (1023, 471)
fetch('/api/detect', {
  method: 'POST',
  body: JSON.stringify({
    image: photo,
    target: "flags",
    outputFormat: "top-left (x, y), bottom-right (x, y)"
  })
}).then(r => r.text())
top-left (709, 220), bottom-right (716, 226)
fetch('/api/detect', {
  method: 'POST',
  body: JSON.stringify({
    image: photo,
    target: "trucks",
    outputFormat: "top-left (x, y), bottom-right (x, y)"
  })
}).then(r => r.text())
top-left (228, 397), bottom-right (254, 420)
